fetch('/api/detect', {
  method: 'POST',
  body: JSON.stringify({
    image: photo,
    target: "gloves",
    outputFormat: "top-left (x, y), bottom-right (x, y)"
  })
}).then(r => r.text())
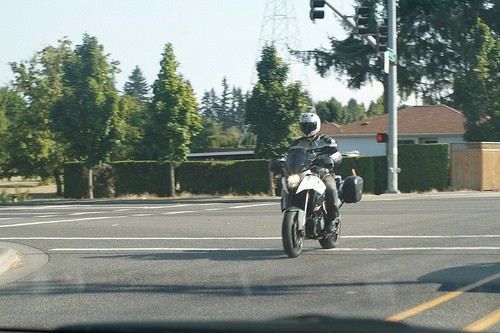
top-left (322, 158), bottom-right (335, 168)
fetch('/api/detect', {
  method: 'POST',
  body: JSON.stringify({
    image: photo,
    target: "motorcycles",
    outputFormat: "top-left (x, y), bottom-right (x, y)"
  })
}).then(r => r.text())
top-left (272, 153), bottom-right (365, 257)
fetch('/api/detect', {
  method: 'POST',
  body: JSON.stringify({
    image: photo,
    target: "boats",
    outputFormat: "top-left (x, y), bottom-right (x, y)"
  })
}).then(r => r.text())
top-left (375, 25), bottom-right (391, 51)
top-left (376, 132), bottom-right (388, 143)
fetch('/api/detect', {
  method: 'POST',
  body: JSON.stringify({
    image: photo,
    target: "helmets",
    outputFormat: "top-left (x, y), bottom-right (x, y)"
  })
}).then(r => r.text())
top-left (298, 112), bottom-right (320, 138)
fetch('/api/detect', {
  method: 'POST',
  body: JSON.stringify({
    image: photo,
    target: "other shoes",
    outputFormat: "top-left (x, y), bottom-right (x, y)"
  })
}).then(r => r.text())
top-left (322, 221), bottom-right (337, 237)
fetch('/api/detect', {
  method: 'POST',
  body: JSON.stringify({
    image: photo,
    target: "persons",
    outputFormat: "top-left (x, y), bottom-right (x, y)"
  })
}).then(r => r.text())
top-left (270, 113), bottom-right (342, 233)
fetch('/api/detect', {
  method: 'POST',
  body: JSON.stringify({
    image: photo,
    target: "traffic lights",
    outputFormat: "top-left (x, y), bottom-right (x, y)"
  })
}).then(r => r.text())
top-left (310, 0), bottom-right (325, 21)
top-left (355, 2), bottom-right (378, 36)
